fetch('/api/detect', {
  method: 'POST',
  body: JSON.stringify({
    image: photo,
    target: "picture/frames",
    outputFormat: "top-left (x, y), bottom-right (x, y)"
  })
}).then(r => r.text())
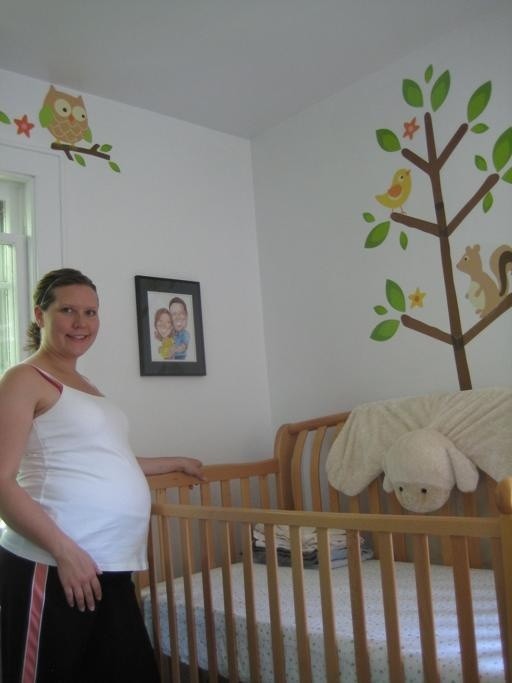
top-left (134, 275), bottom-right (207, 377)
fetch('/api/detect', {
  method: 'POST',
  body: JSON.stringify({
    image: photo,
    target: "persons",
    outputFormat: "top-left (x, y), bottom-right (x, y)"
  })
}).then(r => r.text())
top-left (155, 297), bottom-right (191, 360)
top-left (0, 268), bottom-right (205, 683)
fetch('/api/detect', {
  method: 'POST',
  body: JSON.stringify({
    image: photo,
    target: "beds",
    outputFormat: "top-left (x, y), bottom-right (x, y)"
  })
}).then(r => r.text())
top-left (135, 389), bottom-right (512, 683)
